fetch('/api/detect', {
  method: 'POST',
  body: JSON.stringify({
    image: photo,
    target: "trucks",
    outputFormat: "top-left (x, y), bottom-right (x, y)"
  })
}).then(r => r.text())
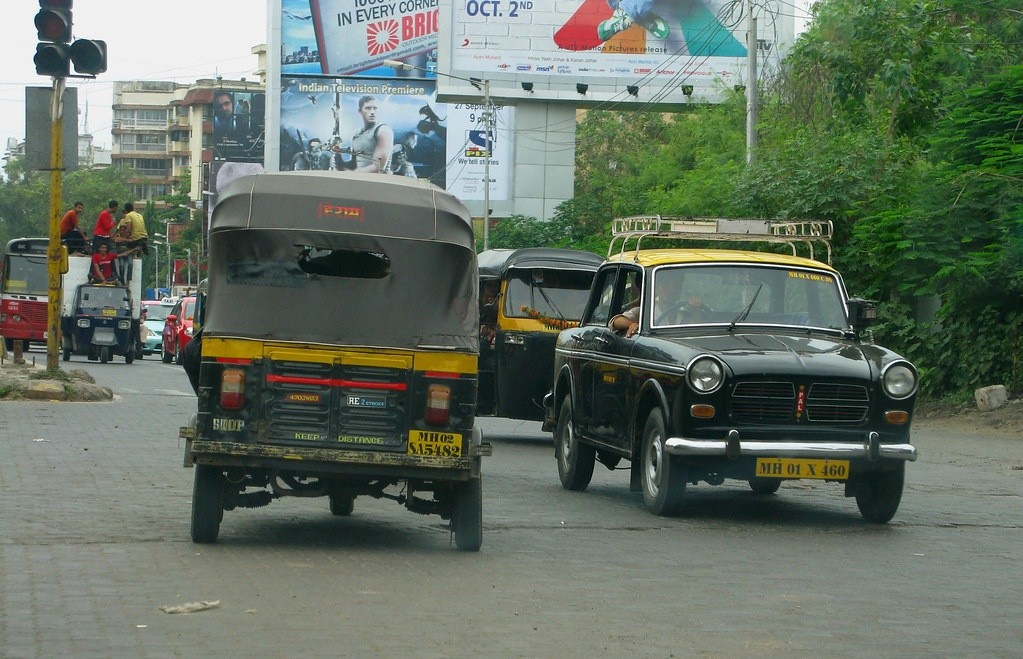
top-left (177, 169), bottom-right (496, 550)
top-left (476, 246), bottom-right (606, 424)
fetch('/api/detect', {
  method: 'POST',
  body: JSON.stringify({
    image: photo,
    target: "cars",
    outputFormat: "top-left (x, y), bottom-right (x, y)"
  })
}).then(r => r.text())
top-left (160, 297), bottom-right (197, 365)
top-left (139, 297), bottom-right (175, 361)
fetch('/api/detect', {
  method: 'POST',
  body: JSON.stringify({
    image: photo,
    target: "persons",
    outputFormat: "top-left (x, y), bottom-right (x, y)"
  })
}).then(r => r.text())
top-left (236, 99), bottom-right (250, 129)
top-left (92, 200), bottom-right (149, 257)
top-left (61, 201), bottom-right (90, 257)
top-left (91, 244), bottom-right (142, 284)
top-left (214, 91), bottom-right (235, 133)
top-left (293, 137), bottom-right (336, 170)
top-left (115, 225), bottom-right (133, 287)
top-left (140, 306), bottom-right (147, 349)
top-left (343, 95), bottom-right (391, 173)
top-left (392, 131), bottom-right (419, 176)
top-left (608, 271), bottom-right (705, 338)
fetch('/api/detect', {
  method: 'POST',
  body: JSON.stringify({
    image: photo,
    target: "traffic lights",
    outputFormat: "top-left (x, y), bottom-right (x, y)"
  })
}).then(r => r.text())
top-left (64, 39), bottom-right (110, 75)
top-left (32, 0), bottom-right (74, 78)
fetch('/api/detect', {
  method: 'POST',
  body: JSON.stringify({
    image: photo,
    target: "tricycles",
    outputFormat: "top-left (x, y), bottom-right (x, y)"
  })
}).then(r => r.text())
top-left (60, 284), bottom-right (136, 364)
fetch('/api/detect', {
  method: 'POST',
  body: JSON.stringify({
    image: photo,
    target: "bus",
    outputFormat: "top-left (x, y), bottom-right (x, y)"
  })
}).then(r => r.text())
top-left (0, 237), bottom-right (57, 351)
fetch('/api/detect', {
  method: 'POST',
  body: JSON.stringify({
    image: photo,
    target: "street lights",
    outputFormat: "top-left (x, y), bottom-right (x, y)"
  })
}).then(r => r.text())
top-left (147, 232), bottom-right (173, 299)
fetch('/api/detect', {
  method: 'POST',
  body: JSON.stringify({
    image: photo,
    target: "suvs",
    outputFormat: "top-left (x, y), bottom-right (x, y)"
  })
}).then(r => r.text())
top-left (539, 212), bottom-right (916, 523)
